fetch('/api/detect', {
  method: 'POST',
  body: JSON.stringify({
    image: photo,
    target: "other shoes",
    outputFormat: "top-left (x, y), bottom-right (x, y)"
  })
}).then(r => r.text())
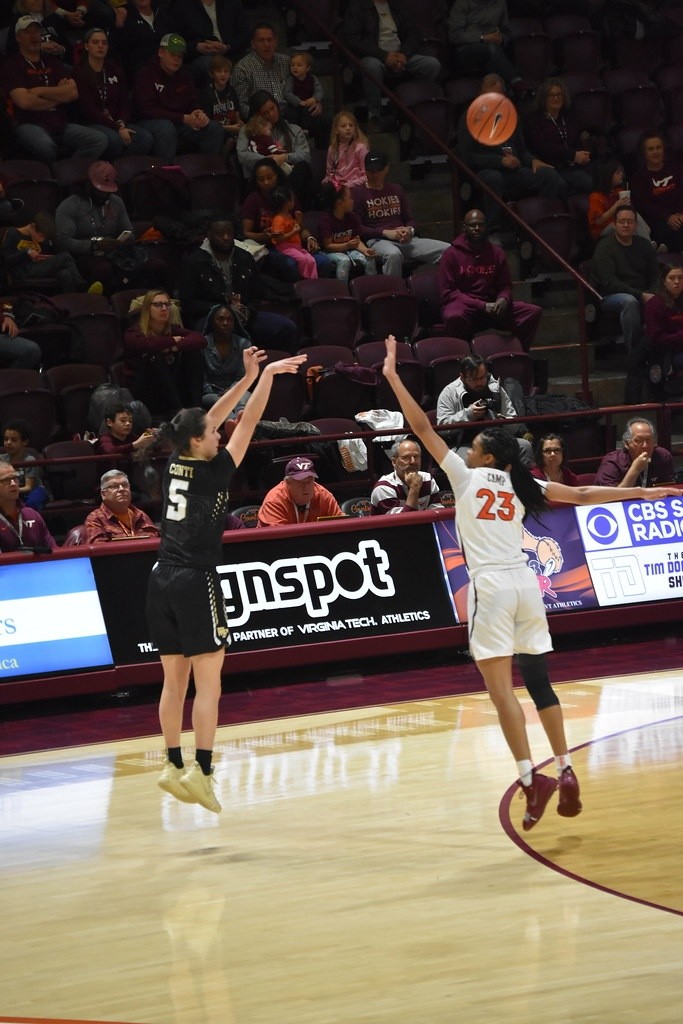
top-left (367, 116), bottom-right (382, 133)
top-left (514, 80), bottom-right (536, 105)
top-left (651, 240), bottom-right (657, 252)
top-left (9, 199), bottom-right (24, 210)
top-left (87, 282), bottom-right (103, 295)
top-left (656, 243), bottom-right (669, 255)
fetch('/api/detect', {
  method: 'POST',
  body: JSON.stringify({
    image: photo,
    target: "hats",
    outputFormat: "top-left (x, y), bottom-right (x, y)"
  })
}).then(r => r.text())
top-left (363, 150), bottom-right (387, 170)
top-left (15, 15), bottom-right (43, 32)
top-left (89, 161), bottom-right (118, 191)
top-left (285, 457), bottom-right (319, 481)
top-left (159, 34), bottom-right (186, 52)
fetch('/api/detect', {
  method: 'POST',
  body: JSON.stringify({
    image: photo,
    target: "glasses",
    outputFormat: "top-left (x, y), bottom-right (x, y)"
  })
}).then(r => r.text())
top-left (464, 222), bottom-right (486, 229)
top-left (464, 375), bottom-right (487, 382)
top-left (149, 302), bottom-right (172, 310)
top-left (102, 483), bottom-right (130, 490)
top-left (542, 447), bottom-right (564, 454)
top-left (628, 434), bottom-right (653, 444)
top-left (394, 454), bottom-right (420, 461)
top-left (546, 93), bottom-right (562, 98)
top-left (0, 474), bottom-right (21, 485)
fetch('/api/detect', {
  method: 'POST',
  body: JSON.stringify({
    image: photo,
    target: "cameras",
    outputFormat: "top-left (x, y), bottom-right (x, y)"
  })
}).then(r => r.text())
top-left (478, 397), bottom-right (497, 410)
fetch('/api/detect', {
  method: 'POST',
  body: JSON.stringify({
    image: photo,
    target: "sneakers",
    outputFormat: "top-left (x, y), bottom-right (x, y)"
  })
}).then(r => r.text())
top-left (517, 768), bottom-right (558, 831)
top-left (179, 761), bottom-right (222, 813)
top-left (557, 766), bottom-right (582, 817)
top-left (158, 759), bottom-right (199, 803)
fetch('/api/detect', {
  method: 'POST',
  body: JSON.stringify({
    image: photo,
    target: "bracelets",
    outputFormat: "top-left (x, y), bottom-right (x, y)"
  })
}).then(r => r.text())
top-left (307, 236), bottom-right (317, 242)
top-left (408, 225), bottom-right (415, 237)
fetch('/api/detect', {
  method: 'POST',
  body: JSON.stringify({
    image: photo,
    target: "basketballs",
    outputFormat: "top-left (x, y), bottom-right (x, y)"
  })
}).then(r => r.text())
top-left (465, 92), bottom-right (518, 147)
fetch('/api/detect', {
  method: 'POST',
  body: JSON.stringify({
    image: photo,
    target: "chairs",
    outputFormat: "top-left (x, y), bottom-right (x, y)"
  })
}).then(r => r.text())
top-left (0, 1), bottom-right (682, 549)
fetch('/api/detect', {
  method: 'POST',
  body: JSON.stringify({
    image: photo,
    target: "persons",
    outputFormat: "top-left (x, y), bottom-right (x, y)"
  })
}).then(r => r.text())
top-left (133, 345), bottom-right (310, 816)
top-left (591, 417), bottom-right (675, 490)
top-left (0, 460), bottom-right (59, 556)
top-left (369, 437), bottom-right (446, 515)
top-left (322, 110), bottom-right (370, 192)
top-left (435, 351), bottom-right (528, 446)
top-left (0, 0), bottom-right (683, 556)
top-left (587, 203), bottom-right (664, 356)
top-left (437, 208), bottom-right (543, 349)
top-left (84, 468), bottom-right (159, 542)
top-left (527, 432), bottom-right (583, 487)
top-left (255, 456), bottom-right (348, 527)
top-left (380, 332), bottom-right (682, 833)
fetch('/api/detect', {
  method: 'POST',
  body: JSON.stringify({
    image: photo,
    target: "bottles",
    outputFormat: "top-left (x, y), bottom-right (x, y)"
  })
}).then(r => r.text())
top-left (358, 510), bottom-right (364, 517)
top-left (404, 337), bottom-right (411, 347)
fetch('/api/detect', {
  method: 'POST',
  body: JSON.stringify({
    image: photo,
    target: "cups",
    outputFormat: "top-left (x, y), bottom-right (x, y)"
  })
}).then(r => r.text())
top-left (619, 191), bottom-right (630, 206)
